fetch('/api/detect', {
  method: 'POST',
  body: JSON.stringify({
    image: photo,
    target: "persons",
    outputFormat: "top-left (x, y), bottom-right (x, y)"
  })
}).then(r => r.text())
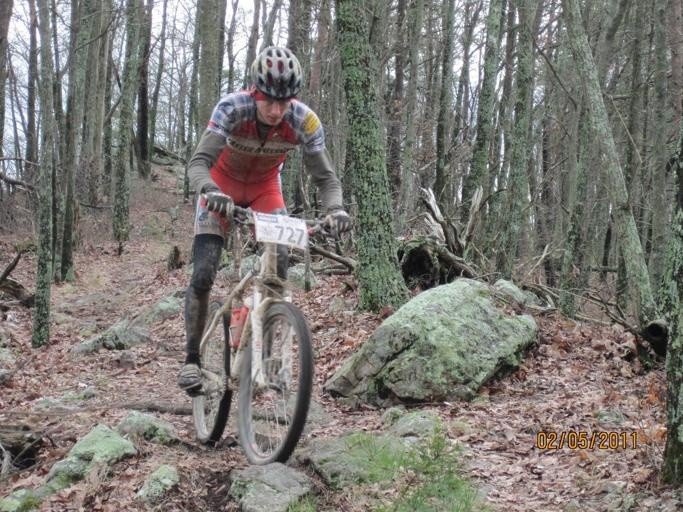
top-left (175, 44), bottom-right (356, 405)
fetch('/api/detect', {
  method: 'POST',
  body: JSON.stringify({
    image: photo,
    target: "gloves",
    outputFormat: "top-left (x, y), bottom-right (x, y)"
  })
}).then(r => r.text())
top-left (204, 185), bottom-right (235, 221)
top-left (322, 204), bottom-right (352, 238)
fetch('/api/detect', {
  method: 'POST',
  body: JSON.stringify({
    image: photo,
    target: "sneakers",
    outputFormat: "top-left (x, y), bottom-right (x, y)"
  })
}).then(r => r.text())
top-left (178, 364), bottom-right (205, 391)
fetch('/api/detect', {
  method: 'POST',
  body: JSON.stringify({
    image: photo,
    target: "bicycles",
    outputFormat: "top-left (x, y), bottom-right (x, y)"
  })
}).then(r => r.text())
top-left (185, 195), bottom-right (358, 467)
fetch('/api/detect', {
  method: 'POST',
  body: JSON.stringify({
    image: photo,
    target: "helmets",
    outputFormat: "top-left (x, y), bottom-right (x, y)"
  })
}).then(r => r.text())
top-left (250, 45), bottom-right (303, 101)
